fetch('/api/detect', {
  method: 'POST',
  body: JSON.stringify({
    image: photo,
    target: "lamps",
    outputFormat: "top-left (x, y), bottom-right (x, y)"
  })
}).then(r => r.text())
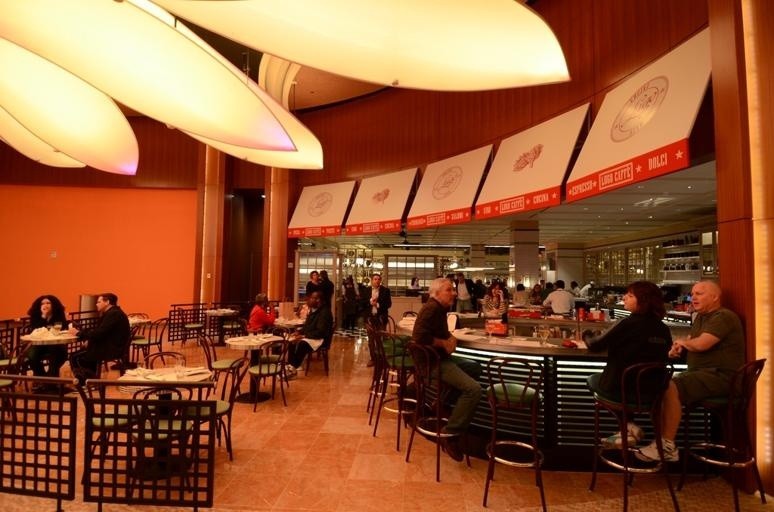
top-left (0, 2), bottom-right (571, 173)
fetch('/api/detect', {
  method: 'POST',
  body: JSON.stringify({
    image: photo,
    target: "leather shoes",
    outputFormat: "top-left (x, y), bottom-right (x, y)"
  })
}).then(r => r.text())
top-left (439, 430), bottom-right (463, 461)
top-left (431, 399), bottom-right (448, 418)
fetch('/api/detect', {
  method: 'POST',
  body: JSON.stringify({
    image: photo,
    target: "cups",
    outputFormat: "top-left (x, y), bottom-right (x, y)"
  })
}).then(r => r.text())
top-left (177, 360), bottom-right (186, 378)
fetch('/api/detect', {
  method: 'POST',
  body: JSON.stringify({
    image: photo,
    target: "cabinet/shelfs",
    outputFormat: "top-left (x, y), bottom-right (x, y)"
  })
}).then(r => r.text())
top-left (659, 234), bottom-right (702, 285)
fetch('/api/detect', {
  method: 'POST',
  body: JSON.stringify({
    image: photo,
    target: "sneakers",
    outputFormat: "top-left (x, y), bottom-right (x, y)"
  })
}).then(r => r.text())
top-left (600, 423), bottom-right (643, 450)
top-left (278, 364), bottom-right (297, 381)
top-left (634, 437), bottom-right (679, 463)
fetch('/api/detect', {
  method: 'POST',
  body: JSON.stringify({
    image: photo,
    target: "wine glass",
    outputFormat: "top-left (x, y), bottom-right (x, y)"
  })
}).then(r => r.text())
top-left (538, 325), bottom-right (550, 345)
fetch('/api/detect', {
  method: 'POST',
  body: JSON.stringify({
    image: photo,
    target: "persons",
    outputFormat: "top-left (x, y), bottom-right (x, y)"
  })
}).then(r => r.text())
top-left (305, 269), bottom-right (371, 333)
top-left (275, 289), bottom-right (333, 381)
top-left (68, 292), bottom-right (131, 395)
top-left (246, 292), bottom-right (276, 333)
top-left (408, 276), bottom-right (420, 289)
top-left (412, 277), bottom-right (483, 462)
top-left (580, 279), bottom-right (673, 447)
top-left (633, 279), bottom-right (746, 463)
top-left (446, 271), bottom-right (597, 319)
top-left (26, 294), bottom-right (68, 391)
top-left (362, 273), bottom-right (393, 367)
